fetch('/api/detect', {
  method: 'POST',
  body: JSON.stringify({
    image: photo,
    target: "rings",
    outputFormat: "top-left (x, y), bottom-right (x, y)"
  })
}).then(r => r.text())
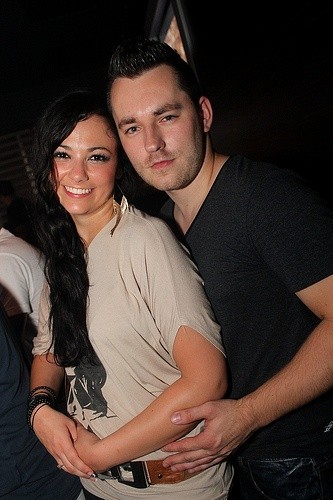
top-left (57, 463), bottom-right (63, 470)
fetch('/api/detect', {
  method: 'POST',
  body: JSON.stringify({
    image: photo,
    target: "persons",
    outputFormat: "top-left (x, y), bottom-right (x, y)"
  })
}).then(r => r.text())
top-left (24, 90), bottom-right (235, 500)
top-left (107, 39), bottom-right (333, 500)
top-left (0, 222), bottom-right (85, 500)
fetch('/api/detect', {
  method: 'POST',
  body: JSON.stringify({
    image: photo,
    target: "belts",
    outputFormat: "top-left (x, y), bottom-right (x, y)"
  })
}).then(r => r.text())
top-left (95, 458), bottom-right (208, 488)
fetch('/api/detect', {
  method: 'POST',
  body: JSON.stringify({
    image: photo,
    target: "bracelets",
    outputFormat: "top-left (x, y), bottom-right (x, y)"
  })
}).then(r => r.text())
top-left (26, 386), bottom-right (58, 432)
